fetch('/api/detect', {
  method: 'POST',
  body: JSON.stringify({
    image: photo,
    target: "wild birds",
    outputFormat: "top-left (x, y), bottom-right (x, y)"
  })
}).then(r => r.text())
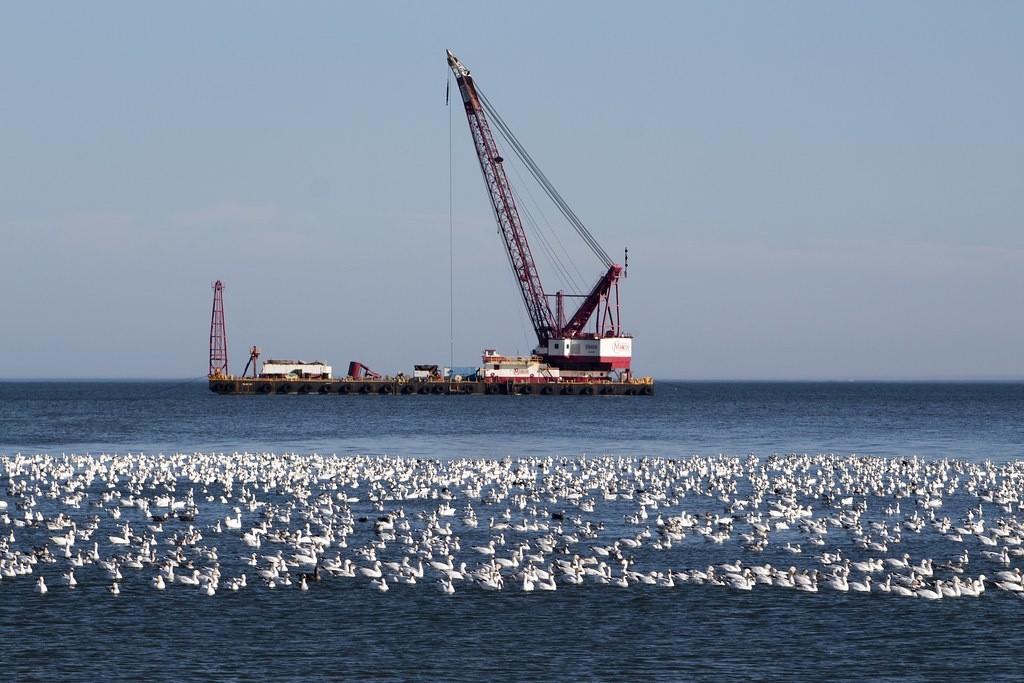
top-left (0, 451), bottom-right (1024, 600)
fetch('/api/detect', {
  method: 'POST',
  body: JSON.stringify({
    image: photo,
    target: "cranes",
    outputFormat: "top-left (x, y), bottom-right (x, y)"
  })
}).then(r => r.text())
top-left (442, 47), bottom-right (657, 383)
